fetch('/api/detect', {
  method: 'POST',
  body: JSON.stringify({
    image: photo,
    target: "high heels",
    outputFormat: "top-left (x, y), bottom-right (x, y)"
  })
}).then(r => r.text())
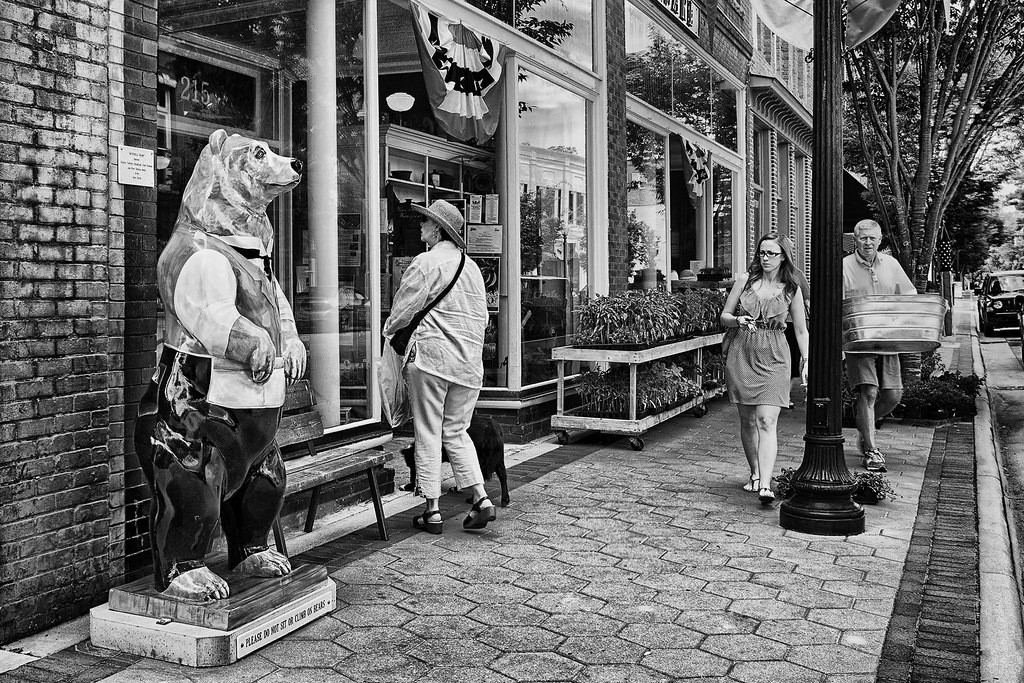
top-left (463, 496), bottom-right (497, 531)
top-left (413, 508), bottom-right (443, 534)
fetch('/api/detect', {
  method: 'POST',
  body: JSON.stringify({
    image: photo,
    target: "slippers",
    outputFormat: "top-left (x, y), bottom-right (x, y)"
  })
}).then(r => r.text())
top-left (742, 474), bottom-right (760, 492)
top-left (758, 488), bottom-right (775, 506)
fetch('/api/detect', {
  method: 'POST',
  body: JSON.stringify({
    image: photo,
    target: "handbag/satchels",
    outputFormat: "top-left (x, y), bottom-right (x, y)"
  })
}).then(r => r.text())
top-left (389, 310), bottom-right (427, 356)
top-left (720, 327), bottom-right (739, 362)
top-left (374, 334), bottom-right (416, 429)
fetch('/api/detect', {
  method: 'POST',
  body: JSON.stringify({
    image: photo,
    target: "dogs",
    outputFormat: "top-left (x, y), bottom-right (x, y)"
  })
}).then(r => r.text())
top-left (400, 417), bottom-right (510, 507)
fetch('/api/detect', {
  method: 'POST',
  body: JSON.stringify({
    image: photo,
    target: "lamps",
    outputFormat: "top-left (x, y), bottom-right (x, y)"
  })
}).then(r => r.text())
top-left (387, 92), bottom-right (415, 112)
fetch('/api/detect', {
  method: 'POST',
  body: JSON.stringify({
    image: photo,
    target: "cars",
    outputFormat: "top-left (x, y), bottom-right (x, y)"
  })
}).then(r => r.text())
top-left (291, 284), bottom-right (371, 333)
top-left (974, 270), bottom-right (1024, 336)
top-left (972, 270), bottom-right (992, 295)
top-left (523, 295), bottom-right (564, 324)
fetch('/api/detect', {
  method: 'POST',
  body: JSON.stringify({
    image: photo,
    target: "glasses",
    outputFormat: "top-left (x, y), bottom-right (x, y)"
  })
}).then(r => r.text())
top-left (757, 251), bottom-right (783, 258)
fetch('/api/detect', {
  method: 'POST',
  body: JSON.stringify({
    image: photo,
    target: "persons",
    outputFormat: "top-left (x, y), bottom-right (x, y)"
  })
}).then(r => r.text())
top-left (383, 195), bottom-right (497, 533)
top-left (843, 219), bottom-right (917, 470)
top-left (720, 231), bottom-right (808, 503)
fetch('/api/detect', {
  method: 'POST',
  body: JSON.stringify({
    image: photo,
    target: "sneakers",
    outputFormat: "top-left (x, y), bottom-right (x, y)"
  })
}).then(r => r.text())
top-left (857, 447), bottom-right (888, 474)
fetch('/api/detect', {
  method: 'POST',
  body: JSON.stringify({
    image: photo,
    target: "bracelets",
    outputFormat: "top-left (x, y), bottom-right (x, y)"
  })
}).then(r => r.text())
top-left (736, 316), bottom-right (740, 328)
top-left (802, 358), bottom-right (807, 363)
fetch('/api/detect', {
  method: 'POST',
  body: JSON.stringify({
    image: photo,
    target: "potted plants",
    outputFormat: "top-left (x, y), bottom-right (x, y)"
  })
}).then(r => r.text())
top-left (569, 288), bottom-right (734, 350)
top-left (772, 464), bottom-right (903, 505)
top-left (573, 351), bottom-right (728, 420)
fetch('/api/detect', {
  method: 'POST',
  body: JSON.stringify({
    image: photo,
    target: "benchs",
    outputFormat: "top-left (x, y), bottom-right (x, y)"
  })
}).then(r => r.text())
top-left (272, 380), bottom-right (394, 557)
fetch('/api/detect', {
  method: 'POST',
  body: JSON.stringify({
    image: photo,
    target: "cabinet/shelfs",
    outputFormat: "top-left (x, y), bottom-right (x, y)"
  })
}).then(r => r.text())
top-left (338, 125), bottom-right (497, 316)
top-left (551, 332), bottom-right (728, 451)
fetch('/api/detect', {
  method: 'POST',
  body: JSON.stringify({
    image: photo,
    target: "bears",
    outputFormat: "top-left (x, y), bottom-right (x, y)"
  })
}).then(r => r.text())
top-left (134, 129), bottom-right (306, 602)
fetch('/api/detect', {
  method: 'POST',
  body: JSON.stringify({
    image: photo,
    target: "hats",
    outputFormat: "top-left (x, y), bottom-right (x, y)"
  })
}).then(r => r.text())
top-left (411, 198), bottom-right (467, 251)
